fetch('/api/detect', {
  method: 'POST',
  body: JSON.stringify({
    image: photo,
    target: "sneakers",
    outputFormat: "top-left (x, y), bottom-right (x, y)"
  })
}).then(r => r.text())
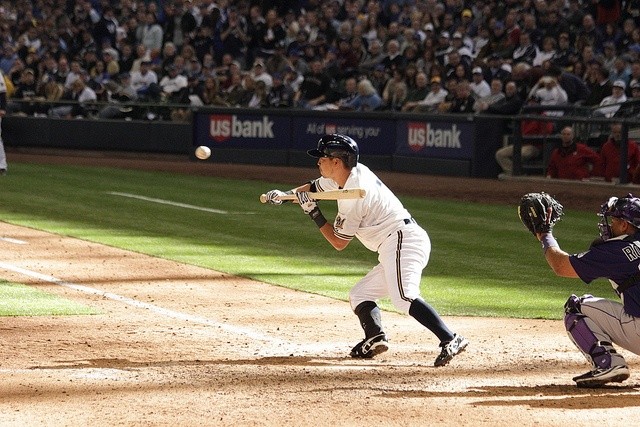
top-left (571, 355), bottom-right (631, 386)
top-left (348, 331), bottom-right (391, 359)
top-left (434, 332), bottom-right (472, 368)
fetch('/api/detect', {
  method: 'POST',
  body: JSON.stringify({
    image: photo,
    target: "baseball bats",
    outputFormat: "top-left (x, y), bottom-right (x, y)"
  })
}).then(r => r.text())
top-left (259, 189), bottom-right (366, 203)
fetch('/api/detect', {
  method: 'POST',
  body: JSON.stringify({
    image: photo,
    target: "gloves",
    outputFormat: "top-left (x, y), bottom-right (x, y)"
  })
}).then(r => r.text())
top-left (265, 189), bottom-right (295, 206)
top-left (296, 190), bottom-right (328, 230)
top-left (529, 198), bottom-right (556, 235)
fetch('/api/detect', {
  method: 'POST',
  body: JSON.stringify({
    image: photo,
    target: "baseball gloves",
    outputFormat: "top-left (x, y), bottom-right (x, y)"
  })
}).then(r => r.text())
top-left (517, 191), bottom-right (564, 237)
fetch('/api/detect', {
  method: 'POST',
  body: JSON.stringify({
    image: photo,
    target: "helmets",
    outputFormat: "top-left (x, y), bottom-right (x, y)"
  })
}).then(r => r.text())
top-left (606, 192), bottom-right (640, 230)
top-left (306, 133), bottom-right (360, 159)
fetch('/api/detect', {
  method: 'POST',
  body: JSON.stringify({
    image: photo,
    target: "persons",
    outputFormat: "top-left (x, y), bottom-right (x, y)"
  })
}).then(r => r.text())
top-left (495, 100), bottom-right (552, 173)
top-left (265, 133), bottom-right (469, 366)
top-left (1, 1), bottom-right (640, 127)
top-left (527, 192), bottom-right (640, 387)
top-left (549, 127), bottom-right (605, 182)
top-left (600, 124), bottom-right (640, 183)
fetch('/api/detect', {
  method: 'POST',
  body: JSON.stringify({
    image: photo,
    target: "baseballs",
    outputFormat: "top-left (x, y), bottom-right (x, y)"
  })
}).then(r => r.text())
top-left (194, 145), bottom-right (212, 160)
